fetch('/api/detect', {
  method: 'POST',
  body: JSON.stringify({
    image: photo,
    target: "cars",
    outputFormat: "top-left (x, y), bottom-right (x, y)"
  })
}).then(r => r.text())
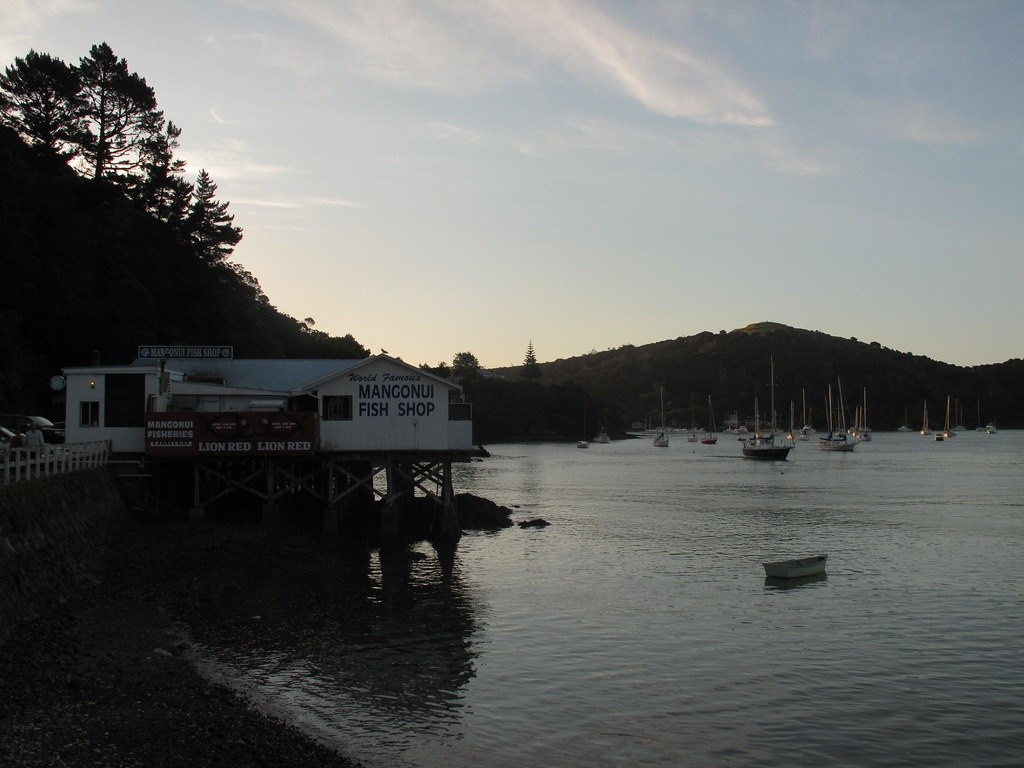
top-left (0, 412), bottom-right (67, 463)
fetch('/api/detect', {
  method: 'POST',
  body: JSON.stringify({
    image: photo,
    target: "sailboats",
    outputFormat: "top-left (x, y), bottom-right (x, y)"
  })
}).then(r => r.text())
top-left (652, 386), bottom-right (672, 447)
top-left (700, 395), bottom-right (718, 445)
top-left (818, 383), bottom-right (858, 452)
top-left (686, 393), bottom-right (699, 442)
top-left (742, 356), bottom-right (791, 464)
top-left (941, 394), bottom-right (984, 437)
top-left (752, 376), bottom-right (870, 443)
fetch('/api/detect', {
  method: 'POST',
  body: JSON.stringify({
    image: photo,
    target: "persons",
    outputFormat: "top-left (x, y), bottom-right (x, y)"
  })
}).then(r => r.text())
top-left (24, 421), bottom-right (46, 471)
top-left (10, 428), bottom-right (24, 475)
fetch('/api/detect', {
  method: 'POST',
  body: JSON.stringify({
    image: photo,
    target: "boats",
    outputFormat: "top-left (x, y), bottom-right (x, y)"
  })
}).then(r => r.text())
top-left (577, 441), bottom-right (589, 448)
top-left (896, 407), bottom-right (913, 432)
top-left (736, 436), bottom-right (746, 441)
top-left (934, 433), bottom-right (944, 441)
top-left (597, 415), bottom-right (611, 443)
top-left (985, 422), bottom-right (997, 435)
top-left (645, 425), bottom-right (749, 435)
top-left (919, 400), bottom-right (931, 435)
top-left (762, 554), bottom-right (828, 578)
